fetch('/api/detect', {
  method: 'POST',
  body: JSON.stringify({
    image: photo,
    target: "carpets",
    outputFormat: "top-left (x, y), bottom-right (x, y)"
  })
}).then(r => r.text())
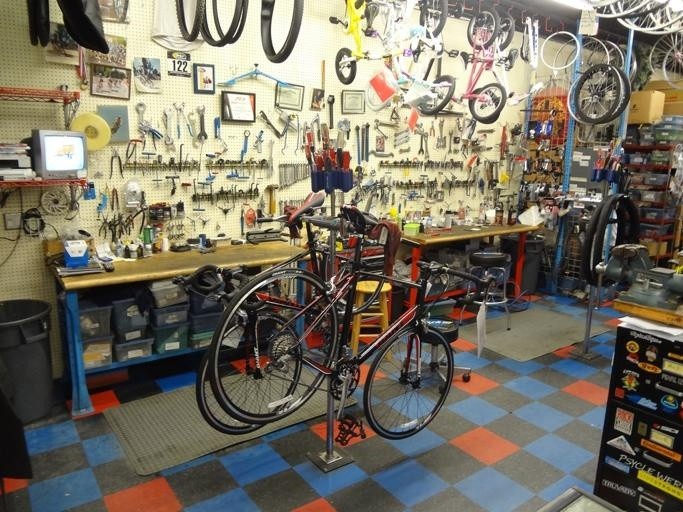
top-left (99, 357), bottom-right (362, 481)
top-left (446, 300), bottom-right (612, 364)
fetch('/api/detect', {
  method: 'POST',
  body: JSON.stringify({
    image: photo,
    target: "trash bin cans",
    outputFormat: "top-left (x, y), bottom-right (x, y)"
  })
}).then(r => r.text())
top-left (0, 298), bottom-right (52, 425)
top-left (499, 235), bottom-right (545, 296)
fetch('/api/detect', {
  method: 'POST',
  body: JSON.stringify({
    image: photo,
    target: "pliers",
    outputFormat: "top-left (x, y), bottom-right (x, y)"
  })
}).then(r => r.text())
top-left (130, 203), bottom-right (148, 235)
top-left (173, 101), bottom-right (194, 140)
top-left (138, 120), bottom-right (164, 139)
top-left (99, 211), bottom-right (134, 243)
top-left (109, 146), bottom-right (124, 180)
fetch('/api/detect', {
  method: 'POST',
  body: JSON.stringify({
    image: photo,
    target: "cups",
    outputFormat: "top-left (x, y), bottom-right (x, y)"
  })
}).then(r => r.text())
top-left (199, 233), bottom-right (206, 245)
top-left (495, 209), bottom-right (503, 224)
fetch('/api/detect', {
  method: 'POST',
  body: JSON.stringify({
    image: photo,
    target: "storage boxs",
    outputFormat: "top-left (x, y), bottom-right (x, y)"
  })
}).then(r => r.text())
top-left (73, 270), bottom-right (276, 374)
top-left (599, 86), bottom-right (683, 274)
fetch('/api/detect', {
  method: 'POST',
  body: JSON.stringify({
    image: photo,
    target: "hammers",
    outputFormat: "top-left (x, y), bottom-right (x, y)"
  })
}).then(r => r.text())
top-left (266, 184), bottom-right (279, 215)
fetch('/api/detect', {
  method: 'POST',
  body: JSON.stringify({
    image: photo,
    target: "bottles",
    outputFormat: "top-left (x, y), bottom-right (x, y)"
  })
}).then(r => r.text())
top-left (425, 320), bottom-right (454, 331)
top-left (458, 202), bottom-right (486, 225)
top-left (143, 223), bottom-right (165, 254)
top-left (507, 204), bottom-right (517, 225)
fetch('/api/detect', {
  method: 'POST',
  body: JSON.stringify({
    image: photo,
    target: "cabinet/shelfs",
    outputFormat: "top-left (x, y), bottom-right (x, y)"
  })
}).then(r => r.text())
top-left (1, 84), bottom-right (89, 212)
top-left (301, 240), bottom-right (394, 351)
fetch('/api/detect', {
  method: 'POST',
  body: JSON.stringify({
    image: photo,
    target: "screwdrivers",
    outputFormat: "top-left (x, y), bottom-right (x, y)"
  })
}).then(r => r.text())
top-left (304, 123), bottom-right (350, 173)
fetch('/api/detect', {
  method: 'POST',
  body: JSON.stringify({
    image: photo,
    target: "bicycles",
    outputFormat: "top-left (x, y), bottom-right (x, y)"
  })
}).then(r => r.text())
top-left (329, 1), bottom-right (517, 124)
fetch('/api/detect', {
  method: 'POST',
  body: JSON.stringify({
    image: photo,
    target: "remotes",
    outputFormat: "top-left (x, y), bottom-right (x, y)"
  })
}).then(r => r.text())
top-left (103, 262), bottom-right (114, 272)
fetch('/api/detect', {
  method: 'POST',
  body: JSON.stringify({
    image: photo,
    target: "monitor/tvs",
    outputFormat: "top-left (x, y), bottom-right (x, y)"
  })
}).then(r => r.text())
top-left (19, 129), bottom-right (88, 181)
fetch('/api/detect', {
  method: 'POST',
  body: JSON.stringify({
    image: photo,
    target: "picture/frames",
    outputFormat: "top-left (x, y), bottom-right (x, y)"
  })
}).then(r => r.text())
top-left (220, 90), bottom-right (258, 124)
top-left (90, 63), bottom-right (132, 101)
top-left (274, 80), bottom-right (305, 112)
top-left (340, 89), bottom-right (366, 115)
top-left (192, 62), bottom-right (216, 96)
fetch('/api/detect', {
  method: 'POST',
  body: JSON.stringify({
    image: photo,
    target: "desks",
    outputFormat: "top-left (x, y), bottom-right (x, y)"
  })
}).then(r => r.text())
top-left (383, 217), bottom-right (548, 331)
top-left (44, 237), bottom-right (320, 416)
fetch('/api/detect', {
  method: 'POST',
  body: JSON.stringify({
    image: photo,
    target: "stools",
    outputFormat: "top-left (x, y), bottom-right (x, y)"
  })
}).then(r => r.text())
top-left (349, 277), bottom-right (395, 364)
